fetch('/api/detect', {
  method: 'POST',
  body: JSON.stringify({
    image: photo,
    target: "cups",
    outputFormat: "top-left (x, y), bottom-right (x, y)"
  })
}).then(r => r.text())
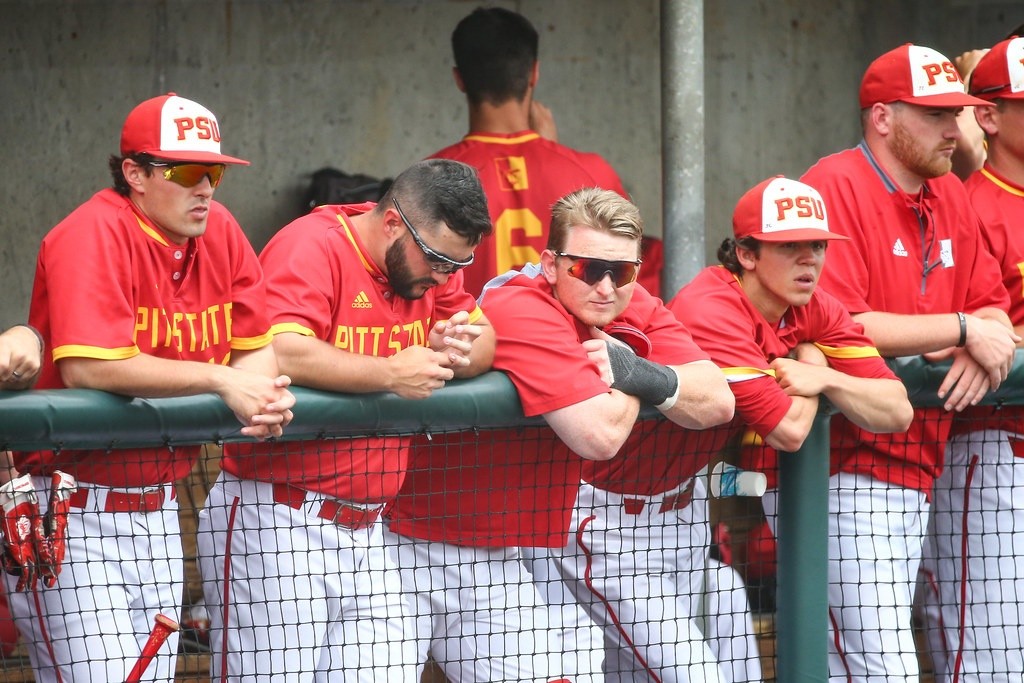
top-left (710, 461), bottom-right (767, 498)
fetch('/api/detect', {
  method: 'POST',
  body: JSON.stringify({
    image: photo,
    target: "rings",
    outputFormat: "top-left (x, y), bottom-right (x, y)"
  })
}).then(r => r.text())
top-left (14, 371), bottom-right (21, 378)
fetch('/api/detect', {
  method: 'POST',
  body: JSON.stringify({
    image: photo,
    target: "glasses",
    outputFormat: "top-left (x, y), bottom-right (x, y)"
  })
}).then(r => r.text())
top-left (144, 159), bottom-right (225, 188)
top-left (392, 196), bottom-right (473, 274)
top-left (550, 250), bottom-right (641, 287)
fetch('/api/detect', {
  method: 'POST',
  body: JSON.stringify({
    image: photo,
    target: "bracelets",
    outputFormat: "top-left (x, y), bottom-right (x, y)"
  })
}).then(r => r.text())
top-left (957, 312), bottom-right (966, 347)
top-left (9, 323), bottom-right (44, 353)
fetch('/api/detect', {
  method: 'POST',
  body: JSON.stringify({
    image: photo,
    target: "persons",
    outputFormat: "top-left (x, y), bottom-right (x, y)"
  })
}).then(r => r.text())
top-left (196, 159), bottom-right (495, 683)
top-left (551, 174), bottom-right (914, 683)
top-left (761, 34), bottom-right (1024, 683)
top-left (383, 187), bottom-right (735, 683)
top-left (0, 92), bottom-right (296, 683)
top-left (428, 6), bottom-right (630, 683)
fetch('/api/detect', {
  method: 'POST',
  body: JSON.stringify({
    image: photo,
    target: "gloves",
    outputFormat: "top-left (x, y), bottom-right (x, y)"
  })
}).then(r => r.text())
top-left (41, 471), bottom-right (75, 587)
top-left (1, 472), bottom-right (52, 592)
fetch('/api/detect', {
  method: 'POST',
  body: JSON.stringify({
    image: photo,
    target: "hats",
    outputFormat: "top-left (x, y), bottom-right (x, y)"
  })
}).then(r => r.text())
top-left (733, 173), bottom-right (849, 241)
top-left (859, 42), bottom-right (995, 109)
top-left (117, 90), bottom-right (251, 166)
top-left (969, 36), bottom-right (1024, 99)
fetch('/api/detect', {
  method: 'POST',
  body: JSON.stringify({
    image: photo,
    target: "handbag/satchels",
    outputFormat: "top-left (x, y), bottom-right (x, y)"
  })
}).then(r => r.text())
top-left (311, 165), bottom-right (391, 205)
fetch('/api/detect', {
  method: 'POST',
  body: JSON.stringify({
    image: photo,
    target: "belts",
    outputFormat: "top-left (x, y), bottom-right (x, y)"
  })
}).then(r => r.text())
top-left (623, 478), bottom-right (698, 514)
top-left (273, 482), bottom-right (379, 531)
top-left (66, 485), bottom-right (177, 509)
top-left (1009, 434), bottom-right (1024, 458)
